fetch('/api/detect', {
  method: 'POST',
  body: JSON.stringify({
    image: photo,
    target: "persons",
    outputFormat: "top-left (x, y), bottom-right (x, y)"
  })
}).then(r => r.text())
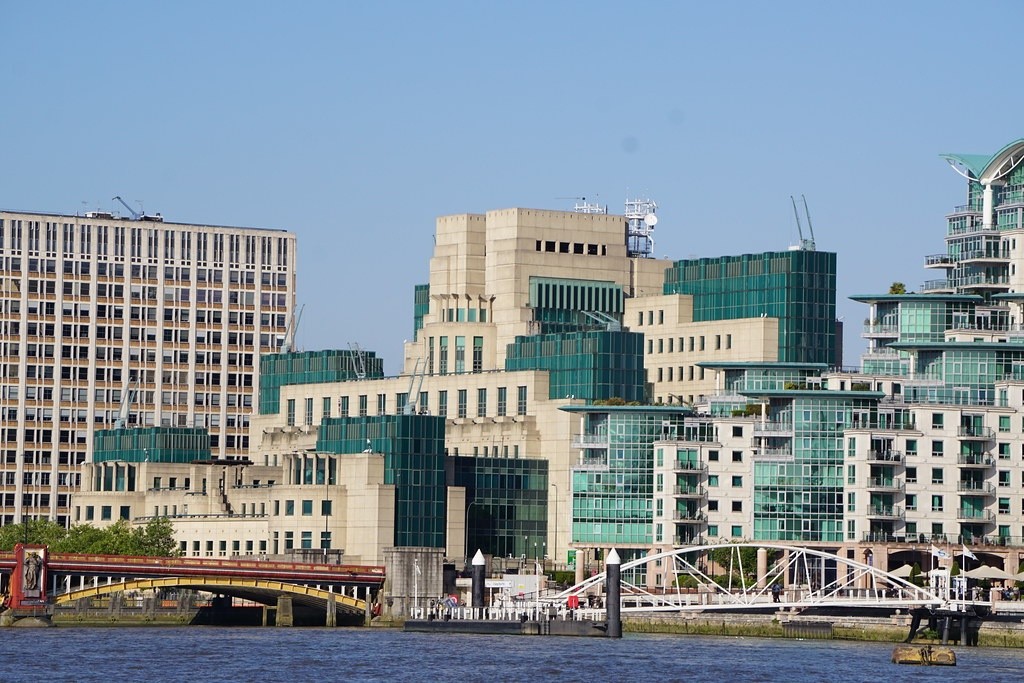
top-left (24, 552), bottom-right (41, 590)
top-left (430, 599), bottom-right (435, 607)
top-left (996, 585), bottom-right (1020, 602)
top-left (772, 583), bottom-right (781, 603)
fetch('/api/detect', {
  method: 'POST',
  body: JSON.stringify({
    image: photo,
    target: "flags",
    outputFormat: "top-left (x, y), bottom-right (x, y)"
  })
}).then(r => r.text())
top-left (931, 545), bottom-right (949, 559)
top-left (963, 545), bottom-right (979, 562)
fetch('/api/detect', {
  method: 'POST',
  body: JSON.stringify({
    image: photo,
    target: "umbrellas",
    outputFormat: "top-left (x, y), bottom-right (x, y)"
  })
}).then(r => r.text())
top-left (875, 563), bottom-right (927, 593)
top-left (1008, 571), bottom-right (1024, 582)
top-left (956, 565), bottom-right (1019, 587)
top-left (913, 565), bottom-right (967, 594)
